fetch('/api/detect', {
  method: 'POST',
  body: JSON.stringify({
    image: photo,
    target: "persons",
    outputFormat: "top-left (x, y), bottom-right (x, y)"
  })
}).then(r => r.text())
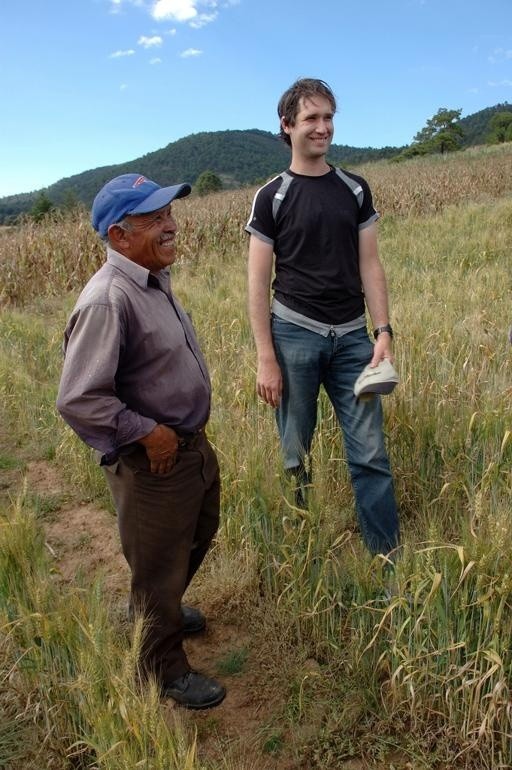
top-left (54, 171), bottom-right (233, 710)
top-left (241, 78), bottom-right (402, 577)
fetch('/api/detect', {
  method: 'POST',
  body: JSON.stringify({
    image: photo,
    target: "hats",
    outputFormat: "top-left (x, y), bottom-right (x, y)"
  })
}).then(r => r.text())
top-left (88, 169), bottom-right (191, 243)
top-left (347, 358), bottom-right (400, 400)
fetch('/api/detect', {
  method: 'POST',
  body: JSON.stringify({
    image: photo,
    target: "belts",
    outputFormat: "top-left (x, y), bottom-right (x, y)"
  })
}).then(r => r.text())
top-left (177, 424), bottom-right (206, 451)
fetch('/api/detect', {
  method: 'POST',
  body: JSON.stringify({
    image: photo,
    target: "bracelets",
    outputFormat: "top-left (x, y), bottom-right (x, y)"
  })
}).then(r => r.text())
top-left (371, 323), bottom-right (393, 341)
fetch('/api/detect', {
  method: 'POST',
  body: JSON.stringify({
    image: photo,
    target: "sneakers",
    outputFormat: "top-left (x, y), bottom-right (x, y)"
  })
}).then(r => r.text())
top-left (156, 671), bottom-right (227, 711)
top-left (179, 604), bottom-right (208, 634)
